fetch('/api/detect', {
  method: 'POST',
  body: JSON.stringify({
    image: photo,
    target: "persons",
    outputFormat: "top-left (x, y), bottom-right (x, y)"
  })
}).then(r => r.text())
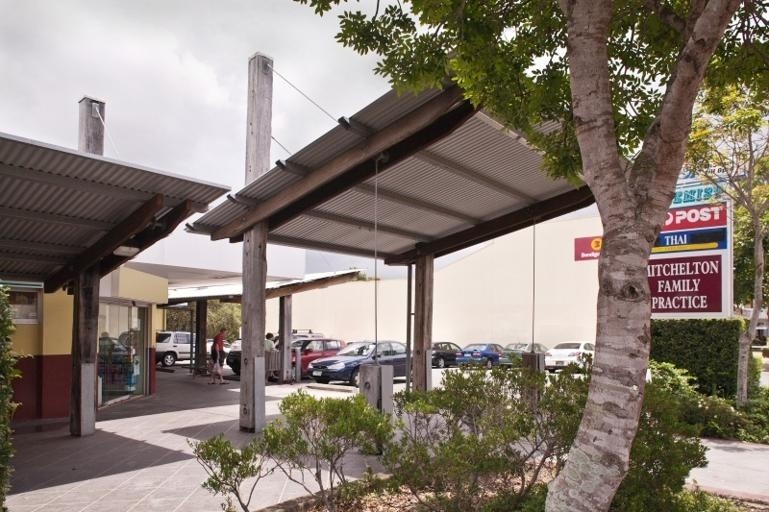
top-left (265, 333), bottom-right (279, 384)
top-left (211, 327), bottom-right (229, 383)
top-left (209, 351), bottom-right (225, 384)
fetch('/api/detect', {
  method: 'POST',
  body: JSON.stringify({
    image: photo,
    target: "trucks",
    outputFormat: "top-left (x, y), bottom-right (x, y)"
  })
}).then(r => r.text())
top-left (755, 326), bottom-right (769, 346)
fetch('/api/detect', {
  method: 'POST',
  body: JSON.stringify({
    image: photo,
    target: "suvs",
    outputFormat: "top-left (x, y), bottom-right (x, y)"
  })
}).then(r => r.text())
top-left (225, 328), bottom-right (325, 381)
top-left (232, 338), bottom-right (242, 349)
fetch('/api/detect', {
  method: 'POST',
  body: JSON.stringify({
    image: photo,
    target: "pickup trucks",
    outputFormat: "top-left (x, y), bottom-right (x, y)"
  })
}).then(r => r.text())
top-left (156, 330), bottom-right (197, 367)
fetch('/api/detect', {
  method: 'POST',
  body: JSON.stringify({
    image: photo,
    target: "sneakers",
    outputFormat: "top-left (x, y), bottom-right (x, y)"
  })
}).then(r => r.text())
top-left (220, 381), bottom-right (230, 384)
top-left (208, 382), bottom-right (218, 384)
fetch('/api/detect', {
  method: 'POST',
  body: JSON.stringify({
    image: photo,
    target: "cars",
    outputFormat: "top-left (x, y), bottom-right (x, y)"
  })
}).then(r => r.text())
top-left (292, 339), bottom-right (345, 381)
top-left (117, 327), bottom-right (133, 349)
top-left (497, 342), bottom-right (549, 371)
top-left (223, 339), bottom-right (230, 348)
top-left (432, 341), bottom-right (463, 370)
top-left (130, 328), bottom-right (138, 354)
top-left (544, 342), bottom-right (595, 372)
top-left (97, 336), bottom-right (129, 371)
top-left (308, 340), bottom-right (413, 387)
top-left (206, 339), bottom-right (214, 345)
top-left (454, 343), bottom-right (504, 369)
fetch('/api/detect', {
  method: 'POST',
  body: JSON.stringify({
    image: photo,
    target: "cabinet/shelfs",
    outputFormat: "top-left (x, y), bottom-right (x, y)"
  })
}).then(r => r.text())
top-left (98, 348), bottom-right (138, 395)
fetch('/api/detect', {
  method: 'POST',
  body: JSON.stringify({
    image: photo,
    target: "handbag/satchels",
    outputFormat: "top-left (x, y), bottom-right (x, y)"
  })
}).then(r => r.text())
top-left (208, 360), bottom-right (220, 374)
